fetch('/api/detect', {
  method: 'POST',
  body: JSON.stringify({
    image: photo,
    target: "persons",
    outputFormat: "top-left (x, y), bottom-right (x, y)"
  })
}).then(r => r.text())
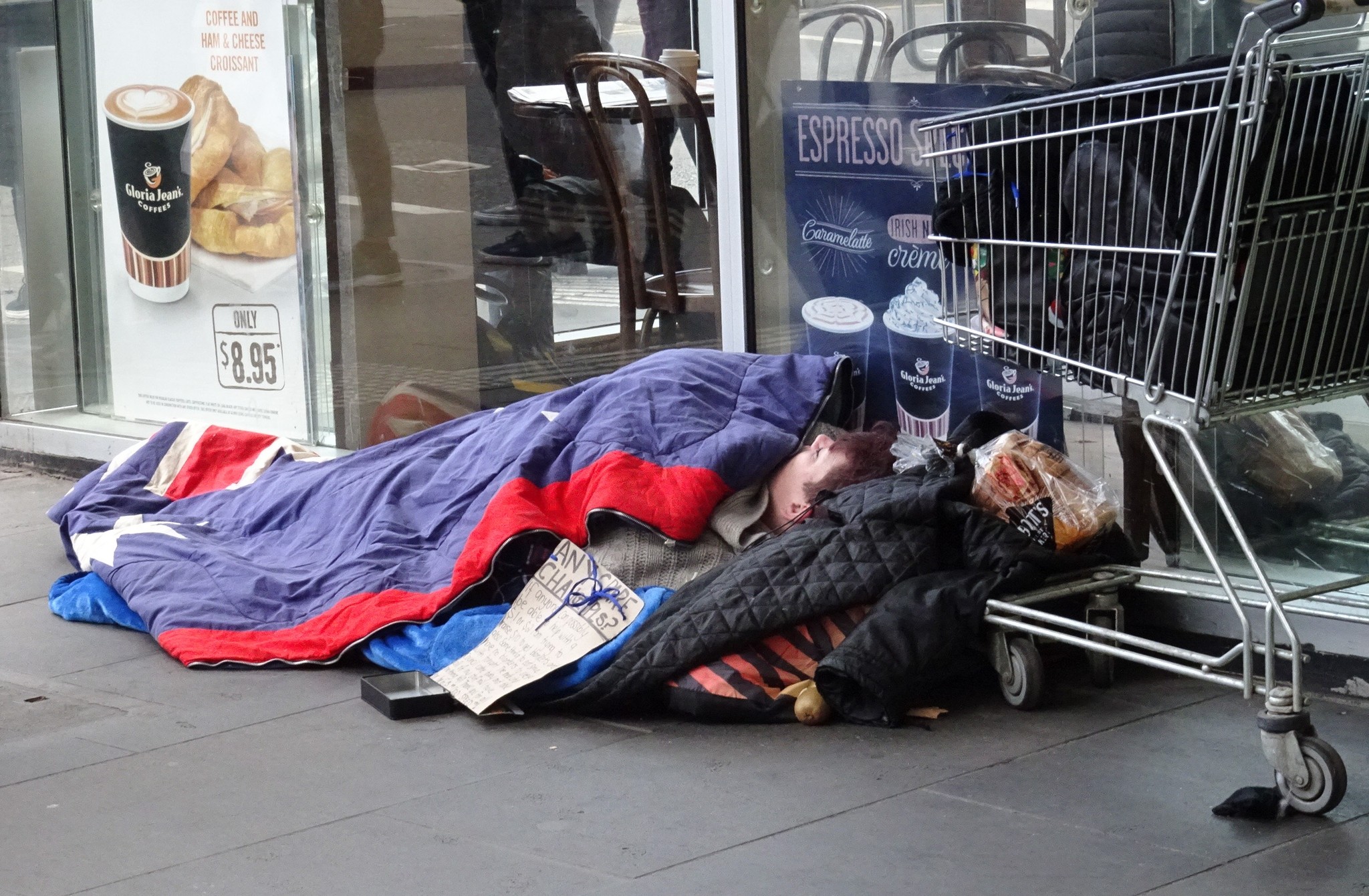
top-left (456, 1), bottom-right (700, 287)
top-left (308, 0), bottom-right (403, 290)
top-left (766, 417), bottom-right (894, 534)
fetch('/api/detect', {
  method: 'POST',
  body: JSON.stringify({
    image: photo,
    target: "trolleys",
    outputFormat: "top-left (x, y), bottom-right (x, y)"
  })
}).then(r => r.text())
top-left (915, 0), bottom-right (1369, 816)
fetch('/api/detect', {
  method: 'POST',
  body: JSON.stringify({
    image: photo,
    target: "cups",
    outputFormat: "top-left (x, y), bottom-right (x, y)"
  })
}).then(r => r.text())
top-left (103, 85), bottom-right (196, 304)
top-left (969, 314), bottom-right (1042, 441)
top-left (802, 296), bottom-right (874, 433)
top-left (882, 309), bottom-right (957, 442)
top-left (659, 48), bottom-right (700, 104)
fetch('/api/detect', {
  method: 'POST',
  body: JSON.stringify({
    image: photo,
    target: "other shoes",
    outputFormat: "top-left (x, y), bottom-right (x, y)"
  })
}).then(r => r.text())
top-left (640, 251), bottom-right (688, 291)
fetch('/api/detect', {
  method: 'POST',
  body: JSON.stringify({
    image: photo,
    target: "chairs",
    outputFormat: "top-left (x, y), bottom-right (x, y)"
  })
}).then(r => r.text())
top-left (564, 51), bottom-right (717, 363)
top-left (799, 2), bottom-right (1079, 92)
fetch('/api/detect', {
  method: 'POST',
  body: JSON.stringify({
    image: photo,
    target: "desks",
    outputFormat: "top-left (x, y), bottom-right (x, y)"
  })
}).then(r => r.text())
top-left (512, 97), bottom-right (713, 365)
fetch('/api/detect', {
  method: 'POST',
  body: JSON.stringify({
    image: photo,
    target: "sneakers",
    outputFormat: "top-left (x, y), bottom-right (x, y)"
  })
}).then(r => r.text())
top-left (321, 239), bottom-right (404, 290)
top-left (474, 200), bottom-right (586, 226)
top-left (481, 231), bottom-right (593, 264)
